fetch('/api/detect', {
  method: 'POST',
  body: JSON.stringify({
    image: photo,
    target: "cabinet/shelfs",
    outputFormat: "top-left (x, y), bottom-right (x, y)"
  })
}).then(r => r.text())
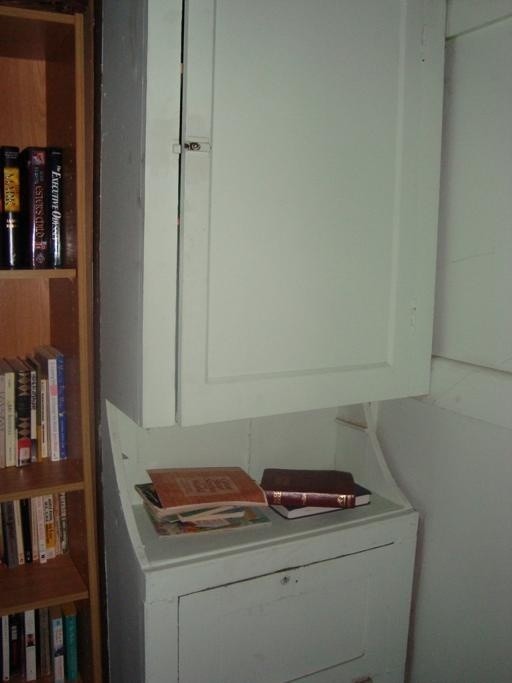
top-left (99, 1), bottom-right (448, 429)
top-left (105, 502), bottom-right (421, 683)
top-left (0, 6), bottom-right (105, 683)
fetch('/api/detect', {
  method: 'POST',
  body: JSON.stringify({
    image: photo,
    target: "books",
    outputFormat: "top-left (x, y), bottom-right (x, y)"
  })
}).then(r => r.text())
top-left (261, 468), bottom-right (372, 520)
top-left (0, 139), bottom-right (68, 270)
top-left (0, 602), bottom-right (78, 683)
top-left (0, 491), bottom-right (68, 566)
top-left (0, 346), bottom-right (67, 468)
top-left (133, 465), bottom-right (271, 535)
